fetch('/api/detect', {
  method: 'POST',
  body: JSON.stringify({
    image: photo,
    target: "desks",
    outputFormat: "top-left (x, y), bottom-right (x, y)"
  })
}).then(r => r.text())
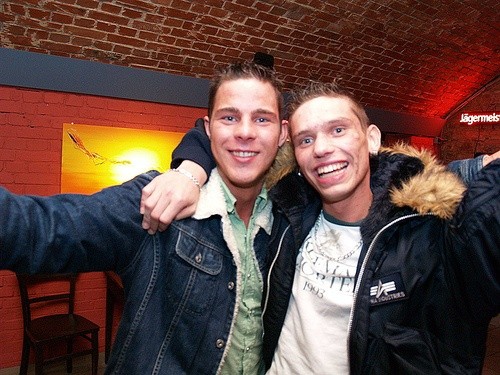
top-left (103, 270), bottom-right (125, 364)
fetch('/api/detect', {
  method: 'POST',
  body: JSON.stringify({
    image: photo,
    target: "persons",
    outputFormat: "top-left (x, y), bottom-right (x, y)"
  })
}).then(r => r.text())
top-left (0, 61), bottom-right (500, 375)
top-left (139, 79), bottom-right (500, 375)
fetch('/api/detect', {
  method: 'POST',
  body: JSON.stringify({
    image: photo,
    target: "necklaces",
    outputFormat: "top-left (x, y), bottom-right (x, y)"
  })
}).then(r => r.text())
top-left (314, 214), bottom-right (362, 261)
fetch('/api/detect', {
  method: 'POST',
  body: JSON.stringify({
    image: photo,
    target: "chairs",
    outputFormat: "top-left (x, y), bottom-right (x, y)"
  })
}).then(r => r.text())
top-left (16, 271), bottom-right (99, 375)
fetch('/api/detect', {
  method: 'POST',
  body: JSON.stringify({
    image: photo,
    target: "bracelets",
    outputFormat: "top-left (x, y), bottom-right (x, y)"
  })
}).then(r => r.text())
top-left (170, 167), bottom-right (203, 195)
